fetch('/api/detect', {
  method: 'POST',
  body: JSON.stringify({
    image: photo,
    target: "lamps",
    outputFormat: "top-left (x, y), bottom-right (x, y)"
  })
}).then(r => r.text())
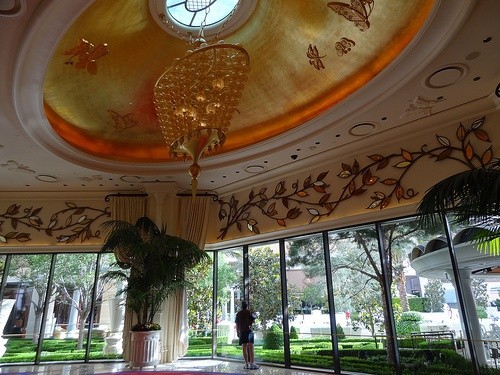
top-left (153, 0), bottom-right (251, 206)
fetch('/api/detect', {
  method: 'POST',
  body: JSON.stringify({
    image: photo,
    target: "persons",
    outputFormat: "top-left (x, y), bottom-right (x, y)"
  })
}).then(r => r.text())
top-left (235, 301), bottom-right (260, 369)
top-left (346, 309), bottom-right (350, 319)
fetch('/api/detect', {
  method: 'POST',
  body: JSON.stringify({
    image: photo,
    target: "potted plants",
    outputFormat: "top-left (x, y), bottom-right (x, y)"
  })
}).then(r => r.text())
top-left (99, 216), bottom-right (213, 371)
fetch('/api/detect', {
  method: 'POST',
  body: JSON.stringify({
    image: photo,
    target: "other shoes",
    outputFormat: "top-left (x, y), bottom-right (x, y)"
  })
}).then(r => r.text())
top-left (250, 363), bottom-right (260, 369)
top-left (243, 363), bottom-right (249, 369)
